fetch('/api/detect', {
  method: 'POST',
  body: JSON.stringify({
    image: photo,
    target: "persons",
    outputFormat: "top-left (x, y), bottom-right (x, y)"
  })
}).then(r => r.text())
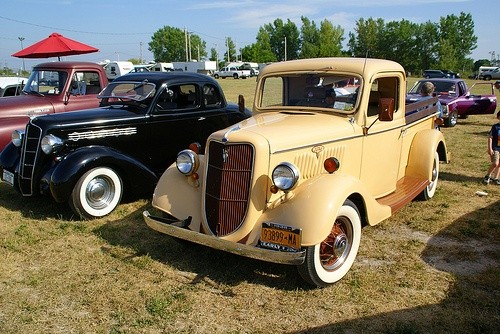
top-left (334, 79), bottom-right (353, 109)
top-left (483, 111), bottom-right (500, 185)
top-left (417, 82), bottom-right (442, 130)
top-left (494, 81), bottom-right (500, 92)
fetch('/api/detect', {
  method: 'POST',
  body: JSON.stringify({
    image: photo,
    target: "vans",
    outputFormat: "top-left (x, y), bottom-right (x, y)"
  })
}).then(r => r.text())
top-left (423, 70), bottom-right (459, 78)
top-left (478, 66), bottom-right (500, 80)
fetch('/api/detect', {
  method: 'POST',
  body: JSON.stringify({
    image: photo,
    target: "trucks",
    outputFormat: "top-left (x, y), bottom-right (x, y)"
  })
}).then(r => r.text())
top-left (97, 61), bottom-right (174, 83)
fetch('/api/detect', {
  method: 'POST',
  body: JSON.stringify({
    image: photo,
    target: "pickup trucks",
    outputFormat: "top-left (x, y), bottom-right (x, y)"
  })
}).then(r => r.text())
top-left (214, 62), bottom-right (260, 79)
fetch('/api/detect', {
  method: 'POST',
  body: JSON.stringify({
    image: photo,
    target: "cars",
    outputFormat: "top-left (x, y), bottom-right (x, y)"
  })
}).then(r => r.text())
top-left (142, 57), bottom-right (451, 289)
top-left (0, 61), bottom-right (137, 153)
top-left (0, 71), bottom-right (252, 219)
top-left (406, 78), bottom-right (497, 127)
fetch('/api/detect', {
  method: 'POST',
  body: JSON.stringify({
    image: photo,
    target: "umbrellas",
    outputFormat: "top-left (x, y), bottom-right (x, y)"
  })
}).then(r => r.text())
top-left (11, 33), bottom-right (100, 61)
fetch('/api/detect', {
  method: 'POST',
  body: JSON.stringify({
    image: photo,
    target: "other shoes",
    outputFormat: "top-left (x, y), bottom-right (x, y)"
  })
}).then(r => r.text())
top-left (484, 175), bottom-right (490, 184)
top-left (492, 179), bottom-right (500, 185)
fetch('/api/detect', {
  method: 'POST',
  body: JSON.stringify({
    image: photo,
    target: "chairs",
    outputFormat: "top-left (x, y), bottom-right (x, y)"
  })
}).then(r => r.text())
top-left (297, 87), bottom-right (335, 107)
top-left (158, 90), bottom-right (178, 110)
top-left (350, 90), bottom-right (385, 116)
